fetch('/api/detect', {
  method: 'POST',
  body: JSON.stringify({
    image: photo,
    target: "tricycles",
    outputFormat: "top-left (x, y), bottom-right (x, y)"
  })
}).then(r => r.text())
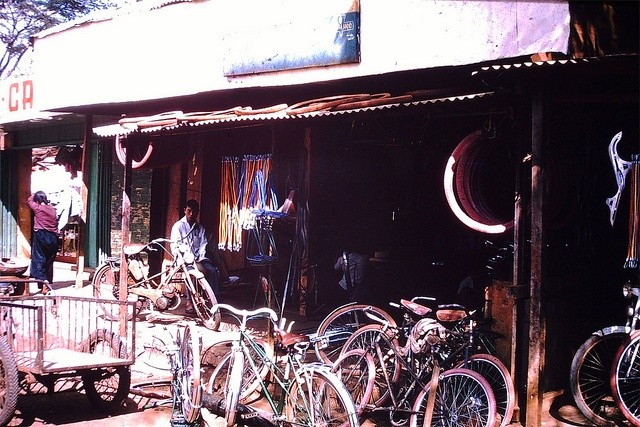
top-left (0, 275), bottom-right (137, 426)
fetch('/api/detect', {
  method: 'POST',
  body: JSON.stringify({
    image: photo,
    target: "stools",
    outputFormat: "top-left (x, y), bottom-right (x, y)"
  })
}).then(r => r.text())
top-left (166, 267), bottom-right (196, 307)
top-left (245, 215), bottom-right (278, 261)
top-left (252, 258), bottom-right (286, 315)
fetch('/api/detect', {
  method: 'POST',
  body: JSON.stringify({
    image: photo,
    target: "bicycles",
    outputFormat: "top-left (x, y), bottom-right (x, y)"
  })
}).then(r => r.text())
top-left (569, 278), bottom-right (640, 426)
top-left (141, 320), bottom-right (203, 426)
top-left (312, 295), bottom-right (518, 426)
top-left (208, 303), bottom-right (361, 427)
top-left (91, 222), bottom-right (221, 330)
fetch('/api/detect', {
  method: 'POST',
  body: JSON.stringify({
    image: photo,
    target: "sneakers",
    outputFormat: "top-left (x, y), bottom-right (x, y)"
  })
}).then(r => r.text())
top-left (185, 308), bottom-right (196, 315)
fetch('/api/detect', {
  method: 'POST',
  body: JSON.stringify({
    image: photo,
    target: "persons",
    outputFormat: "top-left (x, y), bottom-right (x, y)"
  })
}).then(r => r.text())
top-left (27, 191), bottom-right (59, 293)
top-left (169, 200), bottom-right (219, 314)
top-left (332, 253), bottom-right (366, 296)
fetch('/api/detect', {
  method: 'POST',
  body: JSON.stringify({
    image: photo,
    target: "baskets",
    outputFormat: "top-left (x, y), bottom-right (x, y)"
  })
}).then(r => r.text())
top-left (257, 214), bottom-right (297, 233)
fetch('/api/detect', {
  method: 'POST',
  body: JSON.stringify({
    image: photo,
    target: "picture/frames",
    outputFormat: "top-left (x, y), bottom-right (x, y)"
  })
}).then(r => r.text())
top-left (222, 0), bottom-right (362, 78)
